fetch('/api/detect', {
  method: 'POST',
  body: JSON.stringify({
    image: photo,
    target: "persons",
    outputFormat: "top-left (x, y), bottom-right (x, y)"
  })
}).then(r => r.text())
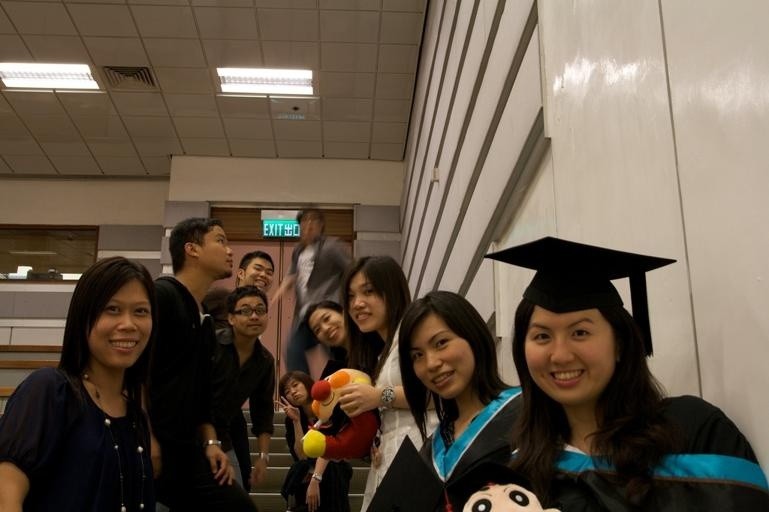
top-left (1, 218), bottom-right (276, 510)
top-left (271, 207), bottom-right (768, 511)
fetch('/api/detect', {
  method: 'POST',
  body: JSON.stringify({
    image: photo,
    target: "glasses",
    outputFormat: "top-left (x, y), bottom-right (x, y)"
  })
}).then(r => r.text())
top-left (231, 307), bottom-right (265, 316)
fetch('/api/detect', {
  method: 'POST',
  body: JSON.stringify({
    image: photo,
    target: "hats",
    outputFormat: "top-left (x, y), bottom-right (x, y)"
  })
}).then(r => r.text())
top-left (484, 236), bottom-right (677, 358)
